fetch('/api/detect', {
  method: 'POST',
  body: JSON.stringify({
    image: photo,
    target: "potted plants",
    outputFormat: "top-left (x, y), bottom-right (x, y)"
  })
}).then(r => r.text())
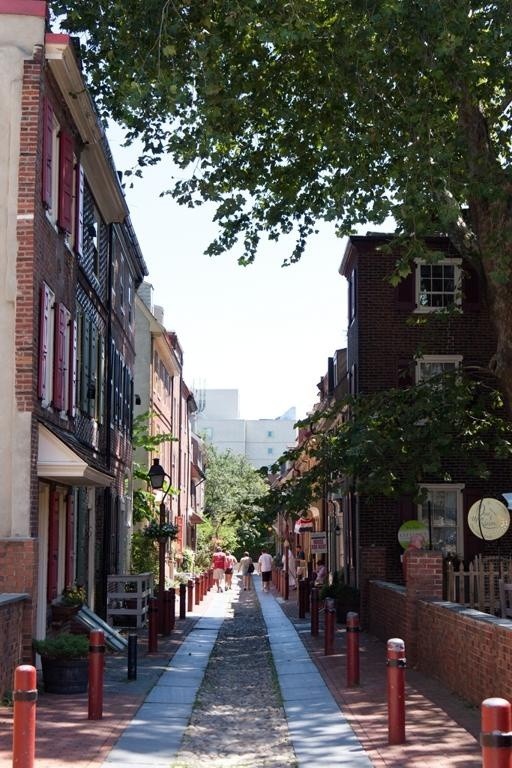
top-left (61, 576), bottom-right (90, 618)
top-left (32, 631), bottom-right (90, 698)
top-left (319, 583), bottom-right (359, 624)
top-left (143, 521), bottom-right (179, 544)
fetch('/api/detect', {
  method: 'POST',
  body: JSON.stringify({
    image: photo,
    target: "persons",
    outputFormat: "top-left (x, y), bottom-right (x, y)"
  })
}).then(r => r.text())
top-left (208, 546), bottom-right (227, 593)
top-left (282, 549), bottom-right (297, 592)
top-left (315, 560), bottom-right (328, 587)
top-left (237, 551), bottom-right (254, 591)
top-left (255, 548), bottom-right (274, 593)
top-left (224, 549), bottom-right (237, 592)
top-left (295, 546), bottom-right (306, 566)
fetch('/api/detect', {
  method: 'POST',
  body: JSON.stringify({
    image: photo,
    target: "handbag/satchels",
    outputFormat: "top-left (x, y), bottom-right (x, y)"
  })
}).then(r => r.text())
top-left (247, 558), bottom-right (255, 574)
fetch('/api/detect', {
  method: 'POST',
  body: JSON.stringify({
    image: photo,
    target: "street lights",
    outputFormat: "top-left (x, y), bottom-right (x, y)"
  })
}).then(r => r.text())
top-left (147, 458), bottom-right (172, 634)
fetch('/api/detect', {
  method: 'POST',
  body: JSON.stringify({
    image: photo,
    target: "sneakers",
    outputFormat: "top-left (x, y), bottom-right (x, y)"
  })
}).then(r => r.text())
top-left (217, 586), bottom-right (269, 594)
